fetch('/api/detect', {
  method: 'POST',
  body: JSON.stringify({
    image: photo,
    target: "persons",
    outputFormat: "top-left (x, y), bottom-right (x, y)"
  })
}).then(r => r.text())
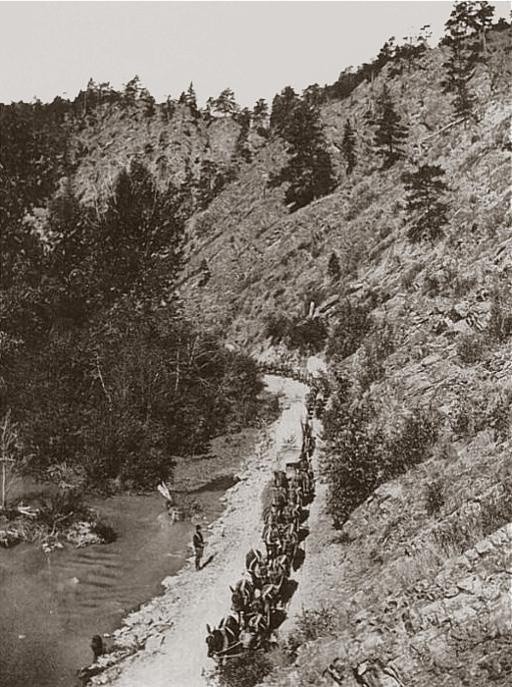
top-left (193, 524), bottom-right (209, 571)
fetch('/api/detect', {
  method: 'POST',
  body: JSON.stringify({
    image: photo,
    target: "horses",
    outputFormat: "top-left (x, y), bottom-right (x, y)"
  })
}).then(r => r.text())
top-left (206, 360), bottom-right (319, 662)
